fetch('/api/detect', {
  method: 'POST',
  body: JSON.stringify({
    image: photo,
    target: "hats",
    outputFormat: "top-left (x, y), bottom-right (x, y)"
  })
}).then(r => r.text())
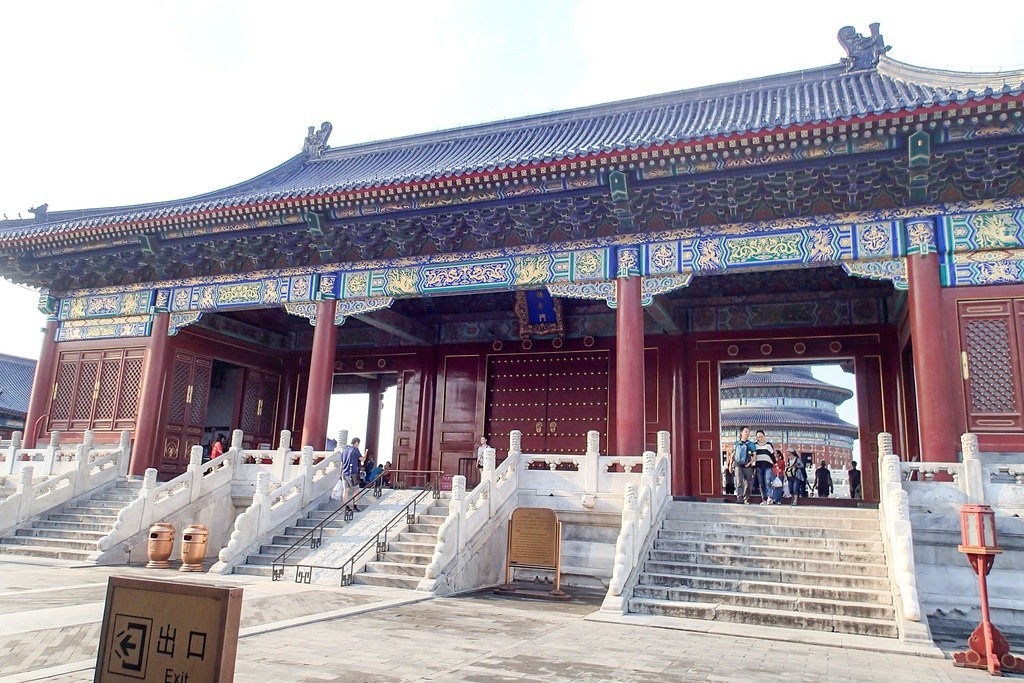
top-left (820, 459), bottom-right (827, 464)
top-left (786, 448), bottom-right (795, 453)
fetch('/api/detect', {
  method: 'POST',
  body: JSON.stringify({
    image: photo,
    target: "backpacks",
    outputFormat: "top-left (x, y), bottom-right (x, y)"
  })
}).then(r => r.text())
top-left (785, 457), bottom-right (796, 478)
top-left (735, 440), bottom-right (751, 465)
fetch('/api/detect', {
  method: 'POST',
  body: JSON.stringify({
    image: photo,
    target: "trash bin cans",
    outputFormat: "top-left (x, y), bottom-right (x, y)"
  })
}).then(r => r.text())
top-left (178, 524), bottom-right (209, 571)
top-left (145, 523), bottom-right (175, 568)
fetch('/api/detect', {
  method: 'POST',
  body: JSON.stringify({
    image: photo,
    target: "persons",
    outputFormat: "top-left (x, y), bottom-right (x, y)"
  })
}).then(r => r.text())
top-left (476, 436), bottom-right (491, 482)
top-left (785, 448), bottom-right (804, 506)
top-left (339, 437), bottom-right (368, 513)
top-left (802, 478), bottom-right (813, 498)
top-left (357, 454), bottom-right (392, 489)
top-left (209, 433), bottom-right (225, 473)
top-left (223, 436), bottom-right (232, 454)
top-left (772, 449), bottom-right (785, 505)
top-left (722, 459), bottom-right (793, 498)
top-left (814, 459), bottom-right (834, 497)
top-left (847, 461), bottom-right (861, 499)
top-left (753, 429), bottom-right (777, 505)
top-left (728, 425), bottom-right (757, 505)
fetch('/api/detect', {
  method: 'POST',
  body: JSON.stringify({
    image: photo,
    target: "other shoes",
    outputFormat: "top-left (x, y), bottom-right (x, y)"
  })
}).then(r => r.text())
top-left (760, 499), bottom-right (766, 505)
top-left (345, 507), bottom-right (351, 511)
top-left (792, 501), bottom-right (797, 505)
top-left (777, 501), bottom-right (781, 504)
top-left (743, 498), bottom-right (751, 505)
top-left (767, 496), bottom-right (774, 505)
top-left (736, 499), bottom-right (743, 503)
top-left (352, 505), bottom-right (360, 512)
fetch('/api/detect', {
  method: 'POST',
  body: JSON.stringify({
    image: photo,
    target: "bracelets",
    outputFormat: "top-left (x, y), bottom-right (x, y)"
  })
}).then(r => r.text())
top-left (775, 465), bottom-right (778, 468)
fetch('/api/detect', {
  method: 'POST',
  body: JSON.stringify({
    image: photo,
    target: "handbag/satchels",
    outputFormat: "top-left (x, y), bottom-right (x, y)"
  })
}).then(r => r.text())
top-left (350, 473), bottom-right (361, 485)
top-left (772, 470), bottom-right (782, 487)
top-left (331, 479), bottom-right (343, 500)
top-left (477, 464), bottom-right (483, 468)
